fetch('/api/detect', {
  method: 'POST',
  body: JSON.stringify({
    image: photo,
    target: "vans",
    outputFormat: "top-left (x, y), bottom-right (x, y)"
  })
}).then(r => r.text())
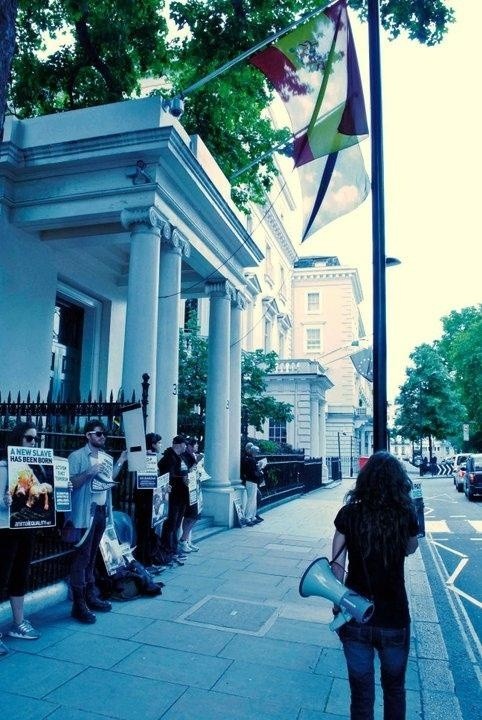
top-left (452, 453), bottom-right (474, 485)
top-left (444, 456), bottom-right (455, 464)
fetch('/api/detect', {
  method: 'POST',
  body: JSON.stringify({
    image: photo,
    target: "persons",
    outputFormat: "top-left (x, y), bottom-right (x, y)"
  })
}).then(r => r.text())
top-left (332, 451), bottom-right (418, 720)
top-left (243, 442), bottom-right (263, 523)
top-left (245, 446), bottom-right (264, 526)
top-left (132, 432), bottom-right (206, 575)
top-left (65, 422), bottom-right (128, 624)
top-left (1, 422), bottom-right (73, 656)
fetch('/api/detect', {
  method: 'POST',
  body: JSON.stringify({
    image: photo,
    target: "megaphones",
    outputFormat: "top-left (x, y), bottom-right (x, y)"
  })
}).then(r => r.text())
top-left (298, 556), bottom-right (376, 632)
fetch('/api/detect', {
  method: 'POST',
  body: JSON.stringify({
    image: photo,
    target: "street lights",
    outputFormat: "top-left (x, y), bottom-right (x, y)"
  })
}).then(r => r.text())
top-left (419, 371), bottom-right (436, 461)
top-left (337, 432), bottom-right (346, 480)
top-left (350, 435), bottom-right (358, 477)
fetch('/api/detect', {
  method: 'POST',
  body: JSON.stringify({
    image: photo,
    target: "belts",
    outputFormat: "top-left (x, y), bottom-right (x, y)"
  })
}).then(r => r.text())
top-left (97, 506), bottom-right (105, 512)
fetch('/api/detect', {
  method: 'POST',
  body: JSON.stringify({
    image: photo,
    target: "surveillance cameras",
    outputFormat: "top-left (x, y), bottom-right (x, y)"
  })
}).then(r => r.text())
top-left (169, 98), bottom-right (185, 116)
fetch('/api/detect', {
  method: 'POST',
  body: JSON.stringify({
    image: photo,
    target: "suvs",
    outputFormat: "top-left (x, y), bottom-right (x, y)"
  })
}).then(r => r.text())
top-left (464, 454), bottom-right (482, 501)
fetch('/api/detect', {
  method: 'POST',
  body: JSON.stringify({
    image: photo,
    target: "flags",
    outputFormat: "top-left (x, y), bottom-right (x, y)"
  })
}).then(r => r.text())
top-left (351, 350), bottom-right (374, 383)
top-left (251, 2), bottom-right (370, 168)
top-left (269, 90), bottom-right (369, 245)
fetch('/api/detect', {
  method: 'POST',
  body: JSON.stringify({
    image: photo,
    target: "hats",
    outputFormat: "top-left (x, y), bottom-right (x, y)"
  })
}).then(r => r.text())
top-left (173, 436), bottom-right (186, 445)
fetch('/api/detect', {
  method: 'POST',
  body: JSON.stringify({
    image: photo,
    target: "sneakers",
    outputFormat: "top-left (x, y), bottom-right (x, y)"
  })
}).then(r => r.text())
top-left (8, 619), bottom-right (40, 640)
top-left (71, 599), bottom-right (96, 623)
top-left (169, 537), bottom-right (200, 562)
top-left (85, 593), bottom-right (112, 612)
top-left (0, 632), bottom-right (9, 656)
top-left (246, 514), bottom-right (264, 527)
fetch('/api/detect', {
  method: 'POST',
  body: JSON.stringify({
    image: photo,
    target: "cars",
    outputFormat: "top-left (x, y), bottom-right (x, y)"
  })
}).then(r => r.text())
top-left (452, 463), bottom-right (465, 492)
top-left (403, 456), bottom-right (420, 468)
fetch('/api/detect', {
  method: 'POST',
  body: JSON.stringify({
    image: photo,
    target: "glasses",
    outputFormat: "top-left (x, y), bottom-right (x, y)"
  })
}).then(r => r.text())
top-left (25, 435), bottom-right (40, 442)
top-left (89, 431), bottom-right (105, 437)
